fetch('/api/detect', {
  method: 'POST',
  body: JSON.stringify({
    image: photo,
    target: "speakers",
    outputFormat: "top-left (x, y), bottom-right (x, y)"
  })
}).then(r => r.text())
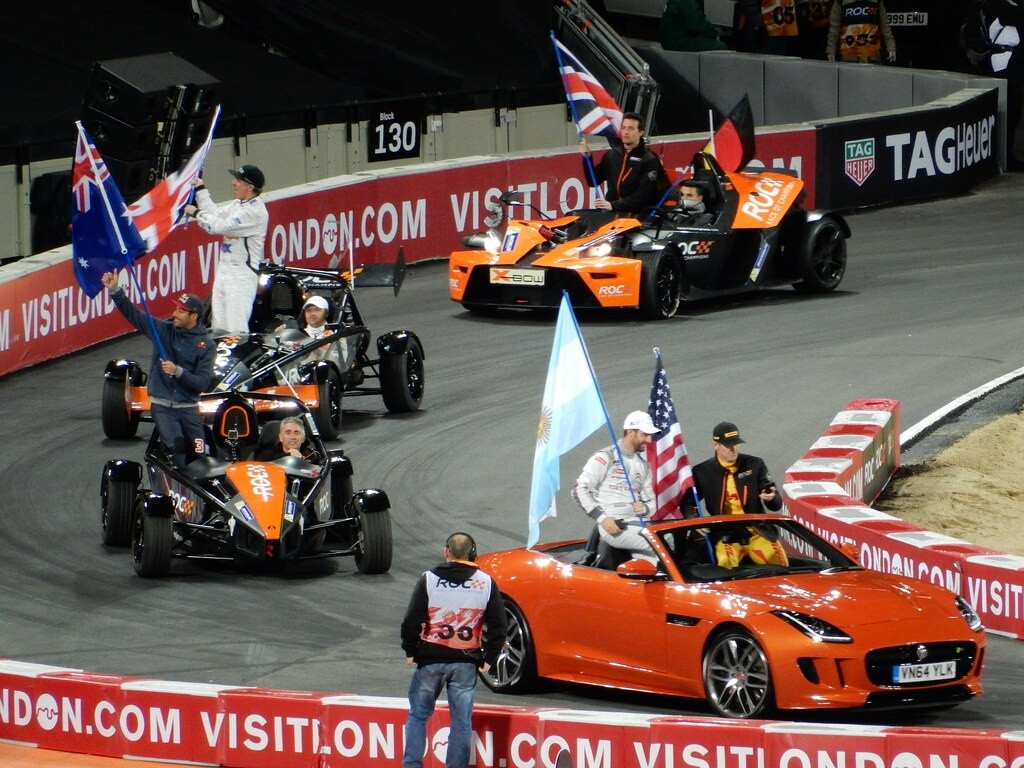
top-left (64, 51), bottom-right (222, 241)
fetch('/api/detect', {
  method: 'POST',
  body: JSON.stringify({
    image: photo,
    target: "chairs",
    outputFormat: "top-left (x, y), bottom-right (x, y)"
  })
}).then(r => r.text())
top-left (251, 420), bottom-right (281, 462)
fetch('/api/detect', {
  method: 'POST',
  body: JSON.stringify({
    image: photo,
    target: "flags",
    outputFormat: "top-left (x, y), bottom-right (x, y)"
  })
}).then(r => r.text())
top-left (630, 345), bottom-right (696, 521)
top-left (687, 94), bottom-right (766, 174)
top-left (527, 289), bottom-right (610, 549)
top-left (63, 103), bottom-right (224, 300)
top-left (551, 33), bottom-right (625, 150)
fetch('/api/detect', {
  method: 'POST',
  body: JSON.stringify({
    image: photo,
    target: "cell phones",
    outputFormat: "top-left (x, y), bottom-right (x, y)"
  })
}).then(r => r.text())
top-left (766, 481), bottom-right (775, 494)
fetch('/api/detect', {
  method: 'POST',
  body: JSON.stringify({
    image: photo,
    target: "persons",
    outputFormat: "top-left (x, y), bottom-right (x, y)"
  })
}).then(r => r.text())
top-left (183, 163), bottom-right (269, 344)
top-left (644, 0), bottom-right (1023, 82)
top-left (652, 180), bottom-right (712, 229)
top-left (568, 409), bottom-right (664, 570)
top-left (272, 296), bottom-right (348, 382)
top-left (259, 416), bottom-right (319, 465)
top-left (101, 268), bottom-right (218, 469)
top-left (398, 533), bottom-right (508, 768)
top-left (692, 421), bottom-right (791, 570)
top-left (579, 112), bottom-right (672, 216)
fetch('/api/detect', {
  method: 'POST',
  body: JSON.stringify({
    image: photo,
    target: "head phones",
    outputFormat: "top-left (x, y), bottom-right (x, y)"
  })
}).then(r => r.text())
top-left (446, 532), bottom-right (477, 562)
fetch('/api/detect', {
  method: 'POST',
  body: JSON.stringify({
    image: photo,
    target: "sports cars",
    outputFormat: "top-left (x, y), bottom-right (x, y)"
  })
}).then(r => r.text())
top-left (100, 392), bottom-right (394, 582)
top-left (445, 160), bottom-right (855, 324)
top-left (398, 512), bottom-right (986, 720)
top-left (97, 262), bottom-right (426, 440)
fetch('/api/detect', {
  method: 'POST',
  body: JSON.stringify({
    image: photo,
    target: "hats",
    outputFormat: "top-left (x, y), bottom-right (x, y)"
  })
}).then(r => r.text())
top-left (713, 422), bottom-right (746, 448)
top-left (624, 410), bottom-right (662, 434)
top-left (304, 296), bottom-right (329, 310)
top-left (229, 165), bottom-right (264, 188)
top-left (171, 293), bottom-right (203, 317)
